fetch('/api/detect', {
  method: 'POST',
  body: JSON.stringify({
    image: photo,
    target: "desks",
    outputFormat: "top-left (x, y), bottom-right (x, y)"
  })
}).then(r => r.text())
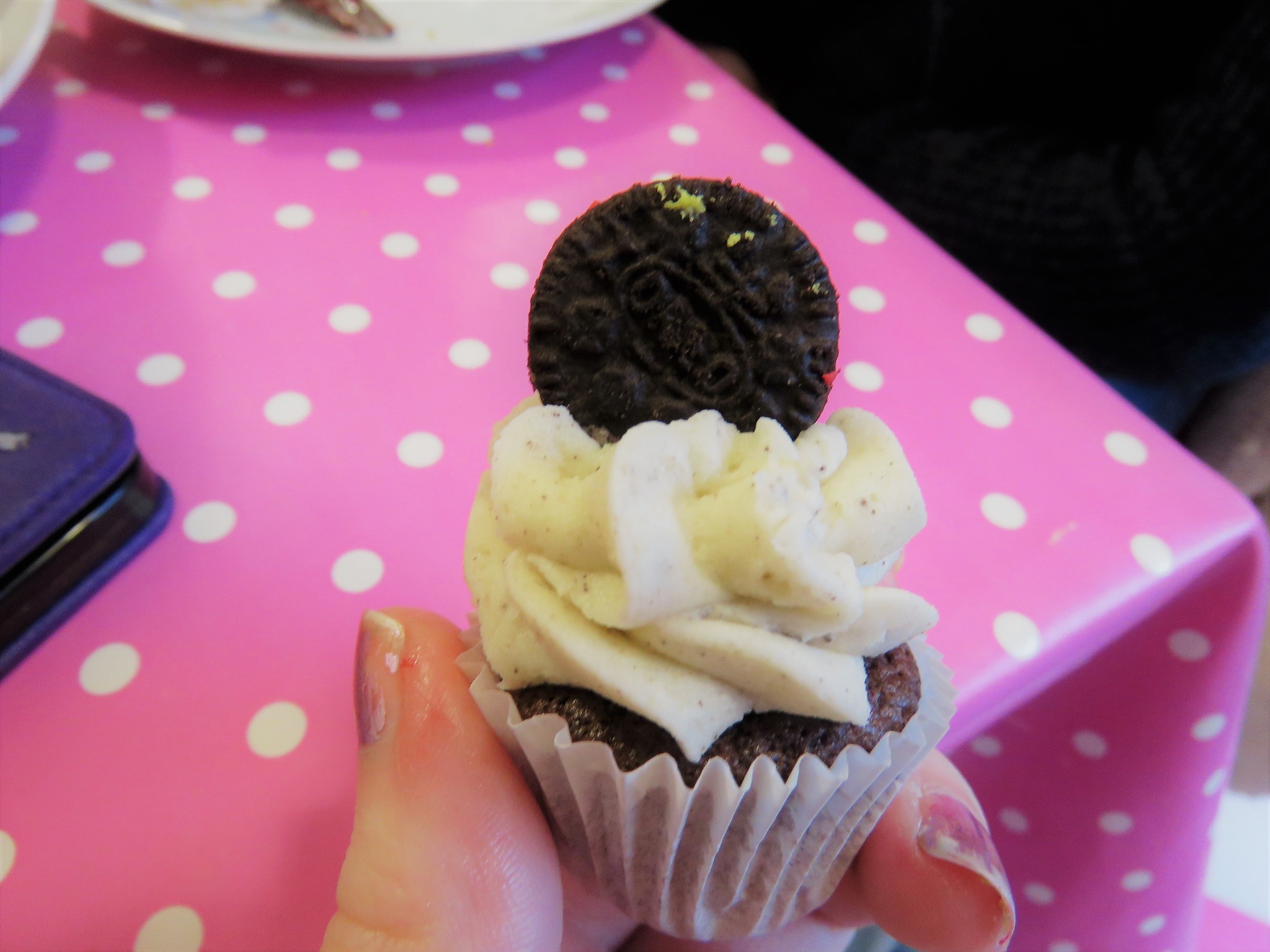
top-left (0, 0), bottom-right (1270, 952)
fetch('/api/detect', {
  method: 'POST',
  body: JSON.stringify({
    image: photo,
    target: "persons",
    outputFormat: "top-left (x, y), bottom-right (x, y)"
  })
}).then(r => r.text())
top-left (342, 609), bottom-right (1015, 952)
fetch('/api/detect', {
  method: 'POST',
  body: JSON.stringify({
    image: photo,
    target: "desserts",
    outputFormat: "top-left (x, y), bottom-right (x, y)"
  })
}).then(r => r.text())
top-left (455, 175), bottom-right (957, 942)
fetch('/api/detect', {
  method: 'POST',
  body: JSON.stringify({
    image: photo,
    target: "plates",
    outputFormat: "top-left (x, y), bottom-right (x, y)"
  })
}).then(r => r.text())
top-left (1, 0), bottom-right (55, 103)
top-left (89, 1), bottom-right (662, 61)
